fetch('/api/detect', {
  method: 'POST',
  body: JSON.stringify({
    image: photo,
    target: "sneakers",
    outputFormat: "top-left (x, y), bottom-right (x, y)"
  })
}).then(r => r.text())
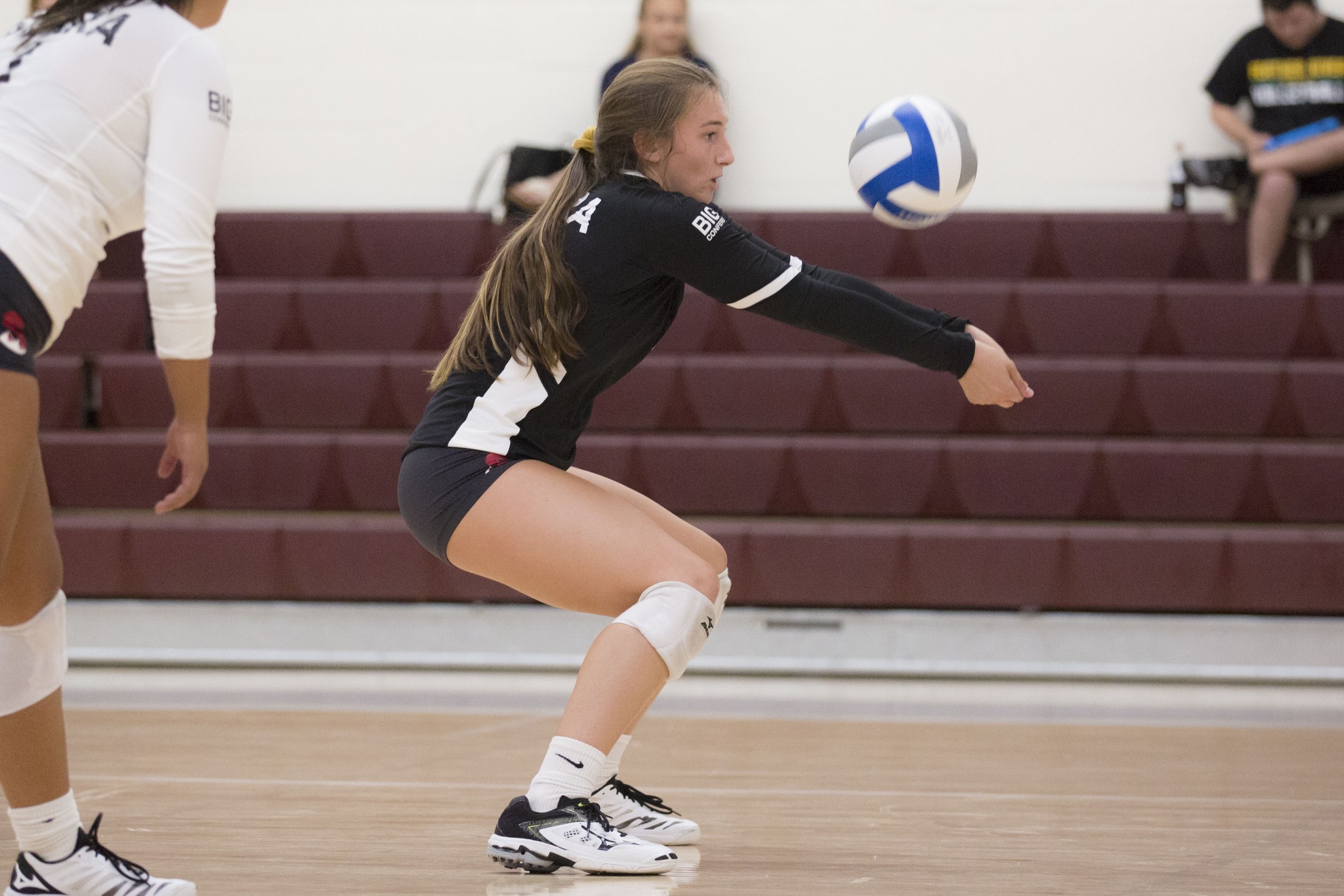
top-left (1, 826), bottom-right (198, 896)
top-left (488, 795), bottom-right (678, 873)
top-left (589, 772), bottom-right (701, 845)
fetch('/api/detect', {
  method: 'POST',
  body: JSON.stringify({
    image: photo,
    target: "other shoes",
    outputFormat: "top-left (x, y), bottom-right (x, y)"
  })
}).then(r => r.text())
top-left (1182, 157), bottom-right (1243, 193)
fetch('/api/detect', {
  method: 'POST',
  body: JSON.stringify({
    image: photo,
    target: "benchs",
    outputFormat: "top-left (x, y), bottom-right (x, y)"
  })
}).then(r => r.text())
top-left (34, 212), bottom-right (1344, 614)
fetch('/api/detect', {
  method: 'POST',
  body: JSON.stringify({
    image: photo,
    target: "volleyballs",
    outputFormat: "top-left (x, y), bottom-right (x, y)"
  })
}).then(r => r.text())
top-left (849, 96), bottom-right (978, 230)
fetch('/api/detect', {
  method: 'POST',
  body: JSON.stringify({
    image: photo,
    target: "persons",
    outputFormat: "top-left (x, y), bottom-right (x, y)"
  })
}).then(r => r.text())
top-left (1202, 0), bottom-right (1343, 286)
top-left (398, 59), bottom-right (1035, 878)
top-left (506, 0), bottom-right (716, 213)
top-left (0, 0), bottom-right (239, 896)
